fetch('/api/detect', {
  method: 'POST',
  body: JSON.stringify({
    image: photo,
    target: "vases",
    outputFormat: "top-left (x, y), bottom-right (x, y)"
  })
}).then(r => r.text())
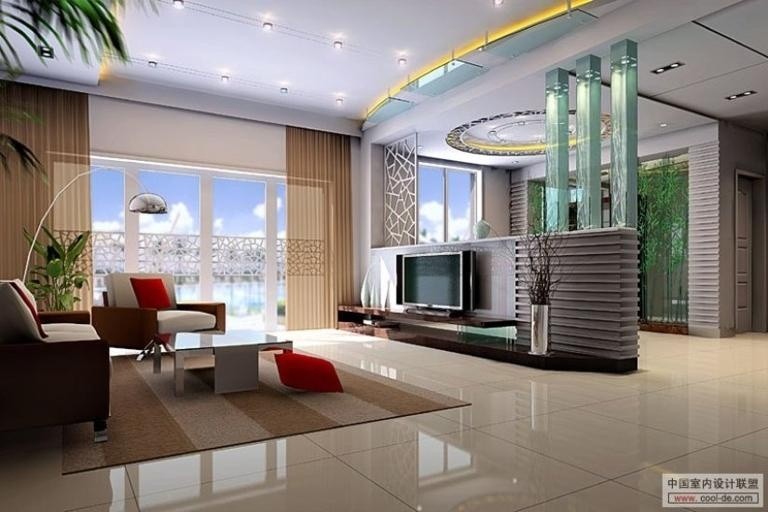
top-left (531, 304), bottom-right (549, 355)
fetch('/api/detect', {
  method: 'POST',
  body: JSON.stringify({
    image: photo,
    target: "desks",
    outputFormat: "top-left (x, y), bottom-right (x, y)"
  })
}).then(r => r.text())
top-left (338, 305), bottom-right (519, 344)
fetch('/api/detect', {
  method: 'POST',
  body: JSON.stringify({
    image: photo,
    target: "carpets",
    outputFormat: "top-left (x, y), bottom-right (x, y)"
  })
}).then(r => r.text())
top-left (62, 350), bottom-right (472, 475)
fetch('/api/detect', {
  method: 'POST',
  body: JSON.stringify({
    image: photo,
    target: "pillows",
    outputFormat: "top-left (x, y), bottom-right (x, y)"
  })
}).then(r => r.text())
top-left (10, 282), bottom-right (48, 339)
top-left (274, 353), bottom-right (344, 393)
top-left (130, 277), bottom-right (172, 309)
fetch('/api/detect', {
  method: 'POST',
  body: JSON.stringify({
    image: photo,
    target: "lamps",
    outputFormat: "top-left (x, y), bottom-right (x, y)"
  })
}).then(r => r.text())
top-left (23, 166), bottom-right (168, 284)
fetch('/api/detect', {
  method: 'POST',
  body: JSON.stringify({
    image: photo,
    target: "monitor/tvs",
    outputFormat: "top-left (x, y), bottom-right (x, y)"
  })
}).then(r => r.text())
top-left (395, 250), bottom-right (478, 319)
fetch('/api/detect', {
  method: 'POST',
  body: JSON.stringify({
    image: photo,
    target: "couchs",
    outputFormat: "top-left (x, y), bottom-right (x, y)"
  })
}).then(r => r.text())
top-left (92, 273), bottom-right (226, 361)
top-left (0, 279), bottom-right (111, 443)
